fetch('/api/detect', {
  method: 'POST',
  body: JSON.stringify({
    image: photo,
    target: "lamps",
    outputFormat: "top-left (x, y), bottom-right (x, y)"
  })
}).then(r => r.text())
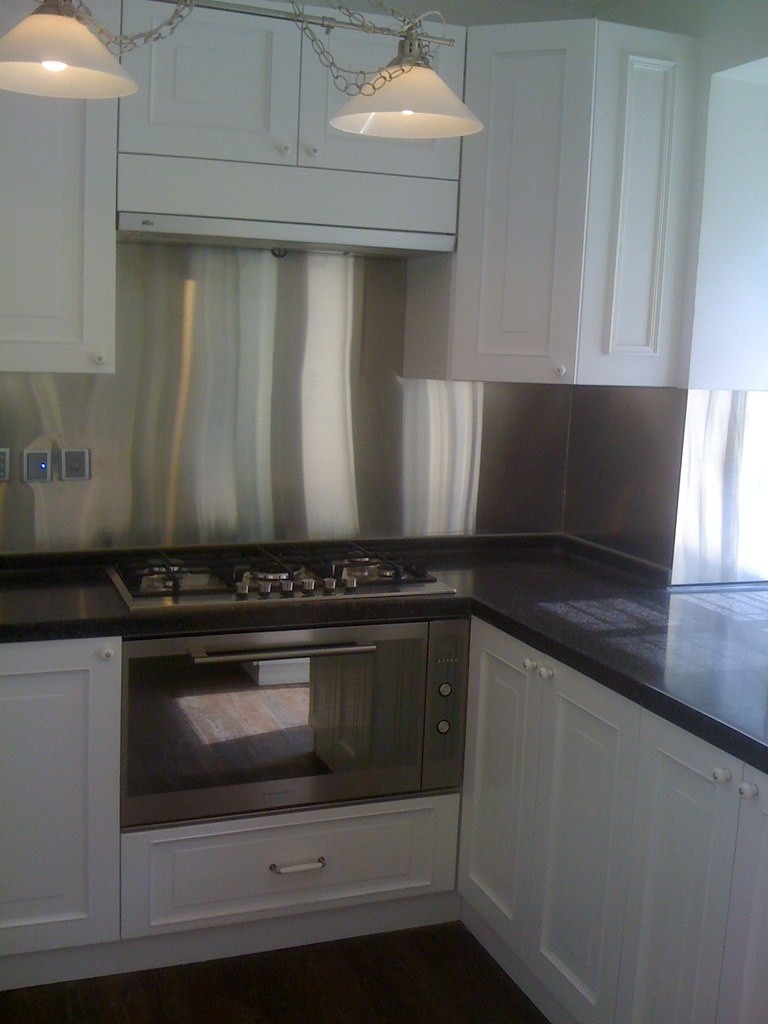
top-left (0, 0), bottom-right (138, 100)
top-left (330, 30), bottom-right (484, 140)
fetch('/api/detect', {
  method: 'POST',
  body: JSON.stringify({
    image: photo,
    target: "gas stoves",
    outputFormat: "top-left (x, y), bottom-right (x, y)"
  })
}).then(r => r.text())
top-left (100, 550), bottom-right (458, 610)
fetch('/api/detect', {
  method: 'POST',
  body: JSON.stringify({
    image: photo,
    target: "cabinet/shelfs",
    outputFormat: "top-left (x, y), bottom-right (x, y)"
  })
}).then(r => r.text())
top-left (121, 0), bottom-right (467, 257)
top-left (451, 20), bottom-right (700, 390)
top-left (618, 700), bottom-right (768, 1024)
top-left (118, 613), bottom-right (468, 978)
top-left (459, 616), bottom-right (644, 1022)
top-left (0, 638), bottom-right (120, 993)
top-left (0, 0), bottom-right (122, 376)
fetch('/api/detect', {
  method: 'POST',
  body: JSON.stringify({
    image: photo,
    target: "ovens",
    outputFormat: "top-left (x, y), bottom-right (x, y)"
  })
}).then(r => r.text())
top-left (120, 615), bottom-right (467, 834)
